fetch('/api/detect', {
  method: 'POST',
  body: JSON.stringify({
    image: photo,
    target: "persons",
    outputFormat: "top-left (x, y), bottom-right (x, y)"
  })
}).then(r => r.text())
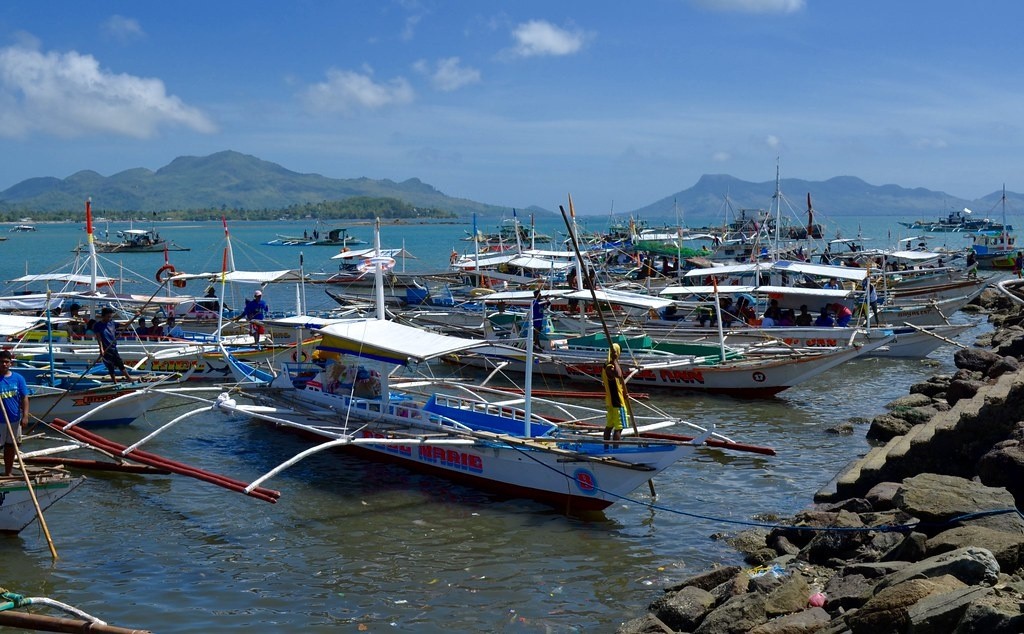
top-left (638, 259), bottom-right (682, 278)
top-left (135, 316), bottom-right (183, 341)
top-left (819, 240), bottom-right (946, 277)
top-left (703, 245), bottom-right (709, 252)
top-left (583, 270), bottom-right (596, 312)
top-left (533, 290), bottom-right (551, 350)
top-left (662, 307), bottom-right (685, 321)
top-left (231, 291), bottom-right (269, 351)
top-left (762, 301), bottom-right (852, 327)
top-left (95, 308), bottom-right (133, 384)
top-left (692, 296), bottom-right (757, 328)
top-left (735, 246), bottom-right (805, 262)
top-left (36, 304), bottom-right (95, 339)
top-left (0, 351), bottom-right (29, 476)
top-left (602, 343), bottom-right (629, 449)
top-left (205, 287), bottom-right (220, 312)
top-left (1015, 251), bottom-right (1023, 278)
top-left (823, 277), bottom-right (838, 289)
top-left (312, 349), bottom-right (344, 370)
top-left (567, 268), bottom-right (579, 314)
top-left (967, 250), bottom-right (978, 280)
top-left (858, 281), bottom-right (879, 327)
top-left (712, 234), bottom-right (722, 249)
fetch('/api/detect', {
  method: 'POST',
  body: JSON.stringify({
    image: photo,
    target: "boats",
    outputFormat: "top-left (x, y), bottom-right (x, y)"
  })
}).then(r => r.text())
top-left (1, 153), bottom-right (1024, 634)
top-left (896, 210), bottom-right (1013, 232)
top-left (70, 219), bottom-right (191, 252)
top-left (64, 215), bottom-right (779, 515)
top-left (266, 226), bottom-right (366, 246)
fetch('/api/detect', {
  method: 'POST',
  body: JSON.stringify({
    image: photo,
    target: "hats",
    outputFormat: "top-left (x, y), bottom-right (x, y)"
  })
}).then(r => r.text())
top-left (207, 288), bottom-right (215, 293)
top-left (255, 290), bottom-right (261, 296)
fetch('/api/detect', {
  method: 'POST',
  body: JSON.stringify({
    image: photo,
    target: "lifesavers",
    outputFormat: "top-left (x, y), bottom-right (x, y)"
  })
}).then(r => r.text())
top-left (290, 349), bottom-right (309, 363)
top-left (449, 251), bottom-right (458, 264)
top-left (155, 265), bottom-right (175, 283)
top-left (355, 255), bottom-right (397, 273)
top-left (470, 287), bottom-right (496, 296)
